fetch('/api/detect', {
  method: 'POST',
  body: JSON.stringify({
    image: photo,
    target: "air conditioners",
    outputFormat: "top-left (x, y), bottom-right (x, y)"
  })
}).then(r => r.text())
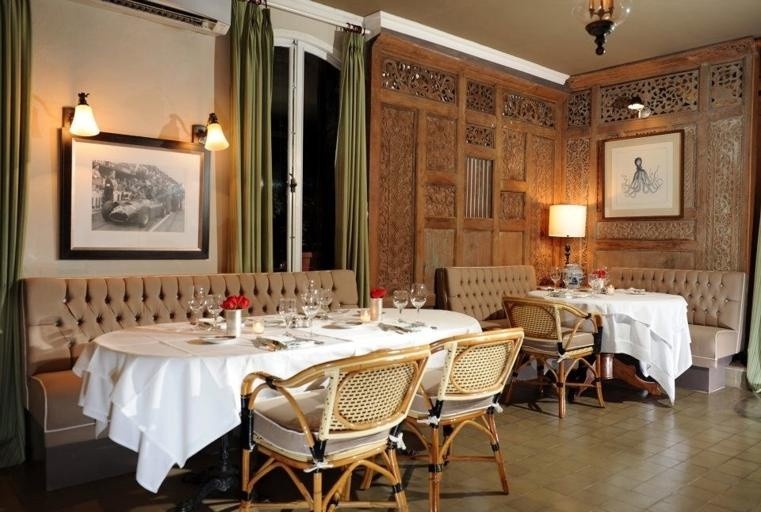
top-left (97, 0), bottom-right (232, 36)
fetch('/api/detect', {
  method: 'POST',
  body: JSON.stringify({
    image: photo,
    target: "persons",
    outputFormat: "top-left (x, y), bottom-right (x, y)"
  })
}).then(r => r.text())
top-left (92, 161), bottom-right (184, 229)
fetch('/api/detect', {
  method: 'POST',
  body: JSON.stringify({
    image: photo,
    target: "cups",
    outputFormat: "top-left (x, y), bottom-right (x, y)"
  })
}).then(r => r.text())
top-left (359, 308), bottom-right (371, 325)
top-left (253, 313), bottom-right (266, 334)
top-left (607, 285), bottom-right (615, 295)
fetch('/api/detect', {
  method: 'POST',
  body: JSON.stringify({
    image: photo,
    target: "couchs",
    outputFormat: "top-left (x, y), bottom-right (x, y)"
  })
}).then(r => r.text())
top-left (434, 265), bottom-right (537, 330)
top-left (19, 269), bottom-right (359, 492)
top-left (608, 266), bottom-right (749, 394)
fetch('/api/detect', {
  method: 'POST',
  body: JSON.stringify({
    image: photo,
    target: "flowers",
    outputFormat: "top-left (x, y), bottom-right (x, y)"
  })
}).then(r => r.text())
top-left (592, 271), bottom-right (605, 278)
top-left (371, 288), bottom-right (385, 298)
top-left (220, 296), bottom-right (250, 309)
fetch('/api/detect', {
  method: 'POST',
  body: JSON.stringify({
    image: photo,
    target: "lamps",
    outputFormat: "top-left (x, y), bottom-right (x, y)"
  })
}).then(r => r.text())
top-left (70, 92), bottom-right (101, 137)
top-left (202, 113), bottom-right (230, 152)
top-left (571, 1), bottom-right (632, 55)
top-left (548, 204), bottom-right (586, 263)
top-left (627, 97), bottom-right (644, 118)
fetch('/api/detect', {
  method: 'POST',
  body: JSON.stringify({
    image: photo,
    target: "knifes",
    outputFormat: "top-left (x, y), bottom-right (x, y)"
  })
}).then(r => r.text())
top-left (245, 318), bottom-right (284, 328)
top-left (299, 312), bottom-right (322, 319)
top-left (579, 289), bottom-right (605, 294)
top-left (200, 335), bottom-right (235, 339)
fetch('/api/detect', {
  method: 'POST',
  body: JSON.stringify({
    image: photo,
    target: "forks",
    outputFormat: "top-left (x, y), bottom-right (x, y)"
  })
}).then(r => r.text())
top-left (377, 321), bottom-right (410, 335)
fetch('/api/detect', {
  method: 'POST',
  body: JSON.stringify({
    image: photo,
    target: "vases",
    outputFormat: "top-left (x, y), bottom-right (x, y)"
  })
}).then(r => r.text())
top-left (563, 263), bottom-right (583, 288)
top-left (370, 298), bottom-right (383, 320)
top-left (224, 309), bottom-right (241, 336)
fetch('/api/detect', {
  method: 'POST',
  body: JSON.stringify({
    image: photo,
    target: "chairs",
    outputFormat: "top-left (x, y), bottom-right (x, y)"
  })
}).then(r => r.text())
top-left (239, 344), bottom-right (432, 512)
top-left (361, 327), bottom-right (525, 512)
top-left (501, 295), bottom-right (606, 418)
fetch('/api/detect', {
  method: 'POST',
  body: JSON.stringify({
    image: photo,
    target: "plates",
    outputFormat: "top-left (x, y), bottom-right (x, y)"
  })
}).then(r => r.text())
top-left (201, 334), bottom-right (235, 344)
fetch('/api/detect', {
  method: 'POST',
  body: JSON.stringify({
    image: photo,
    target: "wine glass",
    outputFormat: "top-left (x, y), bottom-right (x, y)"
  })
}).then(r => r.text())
top-left (392, 282), bottom-right (428, 328)
top-left (586, 273), bottom-right (600, 299)
top-left (278, 278), bottom-right (336, 343)
top-left (549, 267), bottom-right (572, 294)
top-left (185, 284), bottom-right (223, 334)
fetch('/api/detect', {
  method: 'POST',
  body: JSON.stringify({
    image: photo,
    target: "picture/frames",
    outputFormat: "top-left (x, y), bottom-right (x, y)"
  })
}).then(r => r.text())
top-left (59, 133), bottom-right (211, 260)
top-left (602, 128), bottom-right (685, 221)
